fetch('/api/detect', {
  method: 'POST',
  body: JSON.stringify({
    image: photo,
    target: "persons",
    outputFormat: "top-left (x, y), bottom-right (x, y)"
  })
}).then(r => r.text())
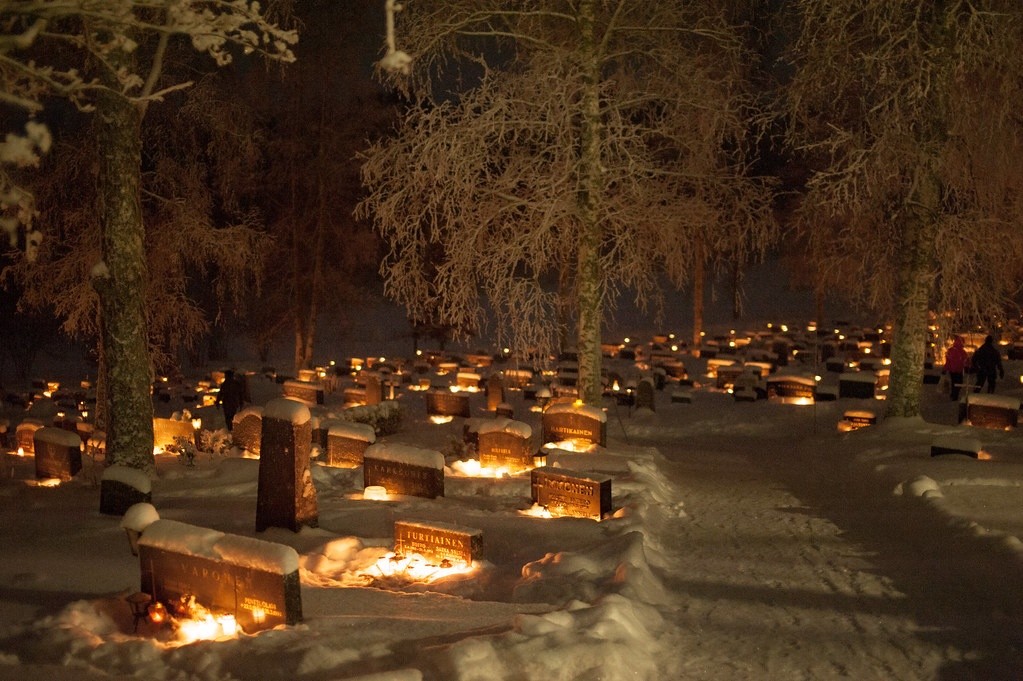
top-left (215, 370), bottom-right (242, 432)
top-left (972, 335), bottom-right (1005, 393)
top-left (943, 336), bottom-right (970, 401)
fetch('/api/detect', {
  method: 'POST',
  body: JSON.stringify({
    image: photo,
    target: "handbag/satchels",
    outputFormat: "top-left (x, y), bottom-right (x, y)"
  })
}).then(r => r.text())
top-left (936, 373), bottom-right (951, 396)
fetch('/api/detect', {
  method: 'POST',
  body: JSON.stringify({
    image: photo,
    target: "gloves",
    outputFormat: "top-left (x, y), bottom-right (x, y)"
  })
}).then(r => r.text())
top-left (238, 402), bottom-right (244, 412)
top-left (215, 402), bottom-right (220, 410)
top-left (941, 369), bottom-right (946, 375)
top-left (999, 369), bottom-right (1005, 379)
